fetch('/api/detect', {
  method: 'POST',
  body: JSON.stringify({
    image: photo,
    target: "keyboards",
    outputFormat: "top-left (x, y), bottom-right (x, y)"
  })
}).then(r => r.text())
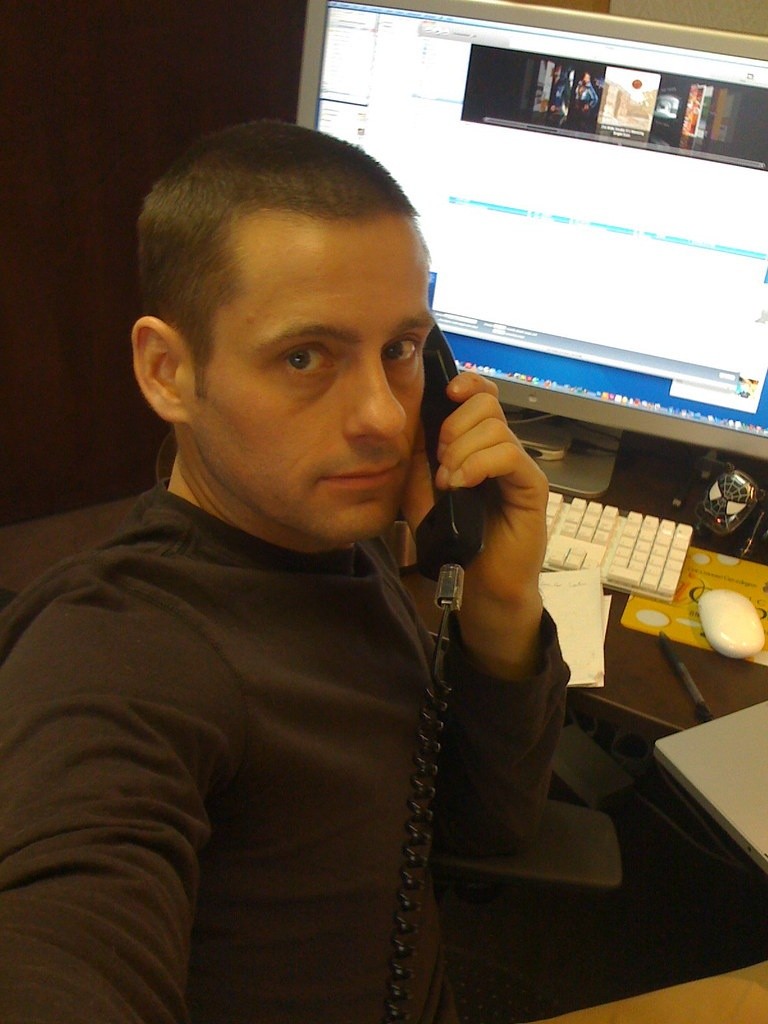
top-left (540, 491), bottom-right (693, 605)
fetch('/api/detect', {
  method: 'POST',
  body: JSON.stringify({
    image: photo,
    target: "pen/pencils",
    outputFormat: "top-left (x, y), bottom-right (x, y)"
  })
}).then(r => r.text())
top-left (655, 624), bottom-right (713, 720)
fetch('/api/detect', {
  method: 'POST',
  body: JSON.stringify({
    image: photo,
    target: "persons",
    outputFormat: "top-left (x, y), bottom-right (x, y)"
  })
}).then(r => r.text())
top-left (550, 63), bottom-right (598, 126)
top-left (0, 116), bottom-right (570, 1024)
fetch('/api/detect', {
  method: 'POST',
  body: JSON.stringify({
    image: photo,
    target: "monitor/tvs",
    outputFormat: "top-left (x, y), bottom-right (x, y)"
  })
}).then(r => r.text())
top-left (298, 0), bottom-right (768, 497)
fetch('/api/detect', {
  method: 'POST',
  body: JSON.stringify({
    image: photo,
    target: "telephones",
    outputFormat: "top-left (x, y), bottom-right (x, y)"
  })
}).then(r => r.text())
top-left (411, 322), bottom-right (484, 575)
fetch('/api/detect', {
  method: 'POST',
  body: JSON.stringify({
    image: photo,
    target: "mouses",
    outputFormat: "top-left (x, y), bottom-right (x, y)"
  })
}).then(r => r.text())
top-left (697, 589), bottom-right (765, 659)
